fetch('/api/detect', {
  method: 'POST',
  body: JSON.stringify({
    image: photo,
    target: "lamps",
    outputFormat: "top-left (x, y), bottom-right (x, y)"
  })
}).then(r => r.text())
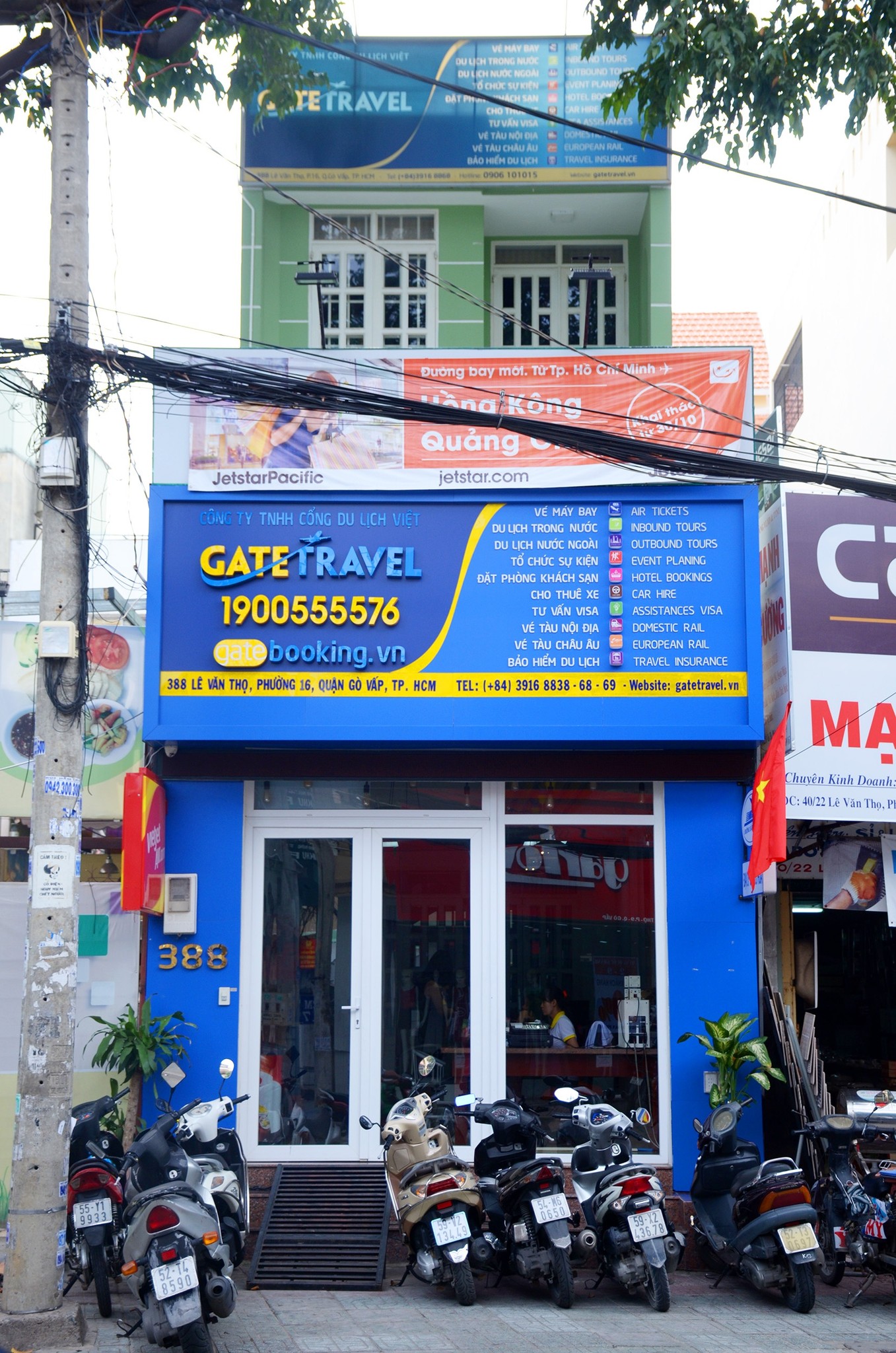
top-left (570, 252), bottom-right (620, 349)
top-left (292, 260), bottom-right (343, 350)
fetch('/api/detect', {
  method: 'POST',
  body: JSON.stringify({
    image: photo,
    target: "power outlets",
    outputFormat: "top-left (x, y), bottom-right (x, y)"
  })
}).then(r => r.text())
top-left (704, 1071), bottom-right (717, 1093)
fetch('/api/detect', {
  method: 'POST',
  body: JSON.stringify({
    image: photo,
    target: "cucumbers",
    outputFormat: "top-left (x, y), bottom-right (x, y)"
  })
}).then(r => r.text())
top-left (14, 624), bottom-right (40, 667)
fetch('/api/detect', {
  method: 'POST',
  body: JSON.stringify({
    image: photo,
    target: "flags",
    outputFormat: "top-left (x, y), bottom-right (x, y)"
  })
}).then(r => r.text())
top-left (747, 699), bottom-right (793, 892)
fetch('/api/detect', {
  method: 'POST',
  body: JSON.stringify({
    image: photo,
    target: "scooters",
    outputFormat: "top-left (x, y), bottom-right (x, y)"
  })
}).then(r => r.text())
top-left (449, 1092), bottom-right (600, 1309)
top-left (549, 1086), bottom-right (687, 1313)
top-left (688, 1096), bottom-right (827, 1314)
top-left (113, 1057), bottom-right (252, 1353)
top-left (358, 1054), bottom-right (507, 1307)
top-left (62, 1086), bottom-right (132, 1318)
top-left (791, 1089), bottom-right (896, 1308)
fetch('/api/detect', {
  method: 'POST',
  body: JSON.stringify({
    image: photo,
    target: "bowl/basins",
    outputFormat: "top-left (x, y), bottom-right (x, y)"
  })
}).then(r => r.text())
top-left (2, 705), bottom-right (36, 770)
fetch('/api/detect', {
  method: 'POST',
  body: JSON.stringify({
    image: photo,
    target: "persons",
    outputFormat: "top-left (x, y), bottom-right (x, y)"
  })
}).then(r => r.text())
top-left (260, 370), bottom-right (343, 468)
top-left (823, 868), bottom-right (876, 909)
top-left (432, 968), bottom-right (469, 1082)
top-left (414, 980), bottom-right (445, 1089)
top-left (518, 985), bottom-right (580, 1134)
top-left (397, 966), bottom-right (418, 1078)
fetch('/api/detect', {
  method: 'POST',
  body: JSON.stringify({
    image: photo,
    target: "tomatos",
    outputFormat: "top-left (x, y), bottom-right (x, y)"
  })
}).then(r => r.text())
top-left (85, 625), bottom-right (129, 669)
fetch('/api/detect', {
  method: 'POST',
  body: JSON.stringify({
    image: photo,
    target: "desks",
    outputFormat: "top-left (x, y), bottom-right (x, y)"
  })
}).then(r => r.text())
top-left (440, 1043), bottom-right (661, 1154)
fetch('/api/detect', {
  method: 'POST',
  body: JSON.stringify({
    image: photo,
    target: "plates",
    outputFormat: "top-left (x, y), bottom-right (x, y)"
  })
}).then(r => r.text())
top-left (80, 699), bottom-right (136, 766)
